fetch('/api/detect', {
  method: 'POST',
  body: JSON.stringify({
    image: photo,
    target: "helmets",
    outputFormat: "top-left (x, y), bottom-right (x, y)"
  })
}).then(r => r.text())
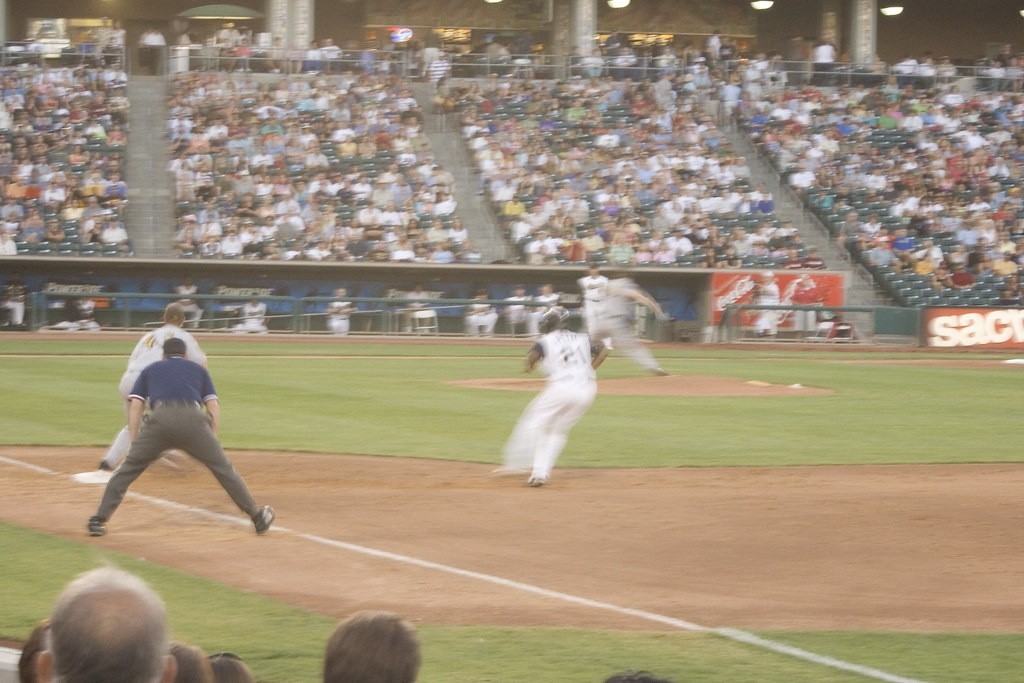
top-left (538, 306), bottom-right (571, 333)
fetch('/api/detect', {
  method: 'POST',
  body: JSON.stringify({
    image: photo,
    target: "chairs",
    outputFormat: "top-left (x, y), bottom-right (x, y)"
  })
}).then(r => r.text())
top-left (0, 46), bottom-right (1024, 340)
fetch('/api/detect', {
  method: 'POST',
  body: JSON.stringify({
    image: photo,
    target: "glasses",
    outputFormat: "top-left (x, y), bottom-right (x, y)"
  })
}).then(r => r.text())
top-left (207, 653), bottom-right (243, 661)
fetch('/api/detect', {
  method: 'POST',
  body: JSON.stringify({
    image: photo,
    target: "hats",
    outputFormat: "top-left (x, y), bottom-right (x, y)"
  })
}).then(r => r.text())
top-left (164, 301), bottom-right (186, 325)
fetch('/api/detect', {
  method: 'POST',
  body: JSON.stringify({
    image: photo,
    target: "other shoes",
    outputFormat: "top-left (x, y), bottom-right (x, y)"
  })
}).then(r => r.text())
top-left (655, 368), bottom-right (668, 376)
top-left (99, 462), bottom-right (113, 472)
top-left (162, 454), bottom-right (184, 469)
top-left (527, 477), bottom-right (545, 486)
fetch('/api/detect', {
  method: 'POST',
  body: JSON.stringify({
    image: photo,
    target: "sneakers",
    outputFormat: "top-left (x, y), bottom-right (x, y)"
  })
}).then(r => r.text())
top-left (253, 505), bottom-right (275, 533)
top-left (88, 517), bottom-right (107, 535)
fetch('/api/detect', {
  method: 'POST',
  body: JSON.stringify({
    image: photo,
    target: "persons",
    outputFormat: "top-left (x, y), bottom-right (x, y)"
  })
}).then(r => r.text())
top-left (494, 305), bottom-right (608, 488)
top-left (99, 303), bottom-right (208, 470)
top-left (0, 17), bottom-right (1024, 376)
top-left (85, 336), bottom-right (275, 537)
top-left (0, 570), bottom-right (666, 683)
